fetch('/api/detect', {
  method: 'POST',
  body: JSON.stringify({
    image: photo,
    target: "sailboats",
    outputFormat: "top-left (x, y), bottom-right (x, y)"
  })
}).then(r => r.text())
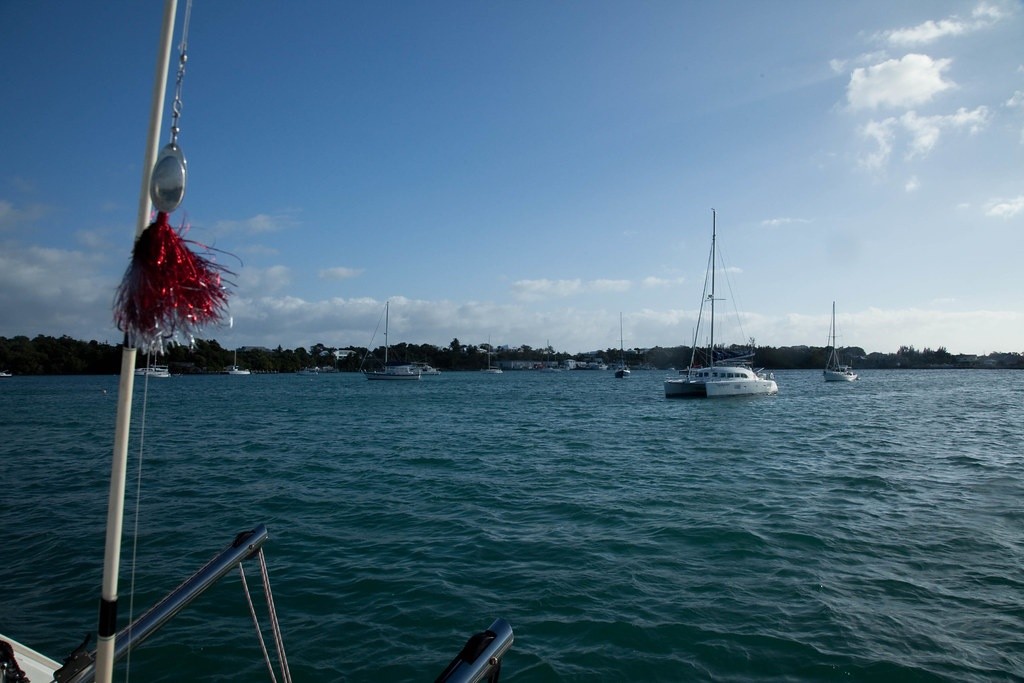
top-left (823, 301), bottom-right (857, 381)
top-left (542, 339), bottom-right (562, 373)
top-left (484, 331), bottom-right (503, 374)
top-left (360, 301), bottom-right (421, 380)
top-left (614, 312), bottom-right (630, 378)
top-left (228, 348), bottom-right (251, 374)
top-left (662, 208), bottom-right (778, 398)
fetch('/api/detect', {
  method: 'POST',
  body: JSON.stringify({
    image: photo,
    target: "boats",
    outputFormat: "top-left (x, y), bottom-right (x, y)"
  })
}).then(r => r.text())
top-left (410, 362), bottom-right (441, 375)
top-left (0, 372), bottom-right (12, 376)
top-left (296, 367), bottom-right (319, 375)
top-left (319, 366), bottom-right (339, 373)
top-left (595, 362), bottom-right (610, 371)
top-left (135, 365), bottom-right (172, 377)
